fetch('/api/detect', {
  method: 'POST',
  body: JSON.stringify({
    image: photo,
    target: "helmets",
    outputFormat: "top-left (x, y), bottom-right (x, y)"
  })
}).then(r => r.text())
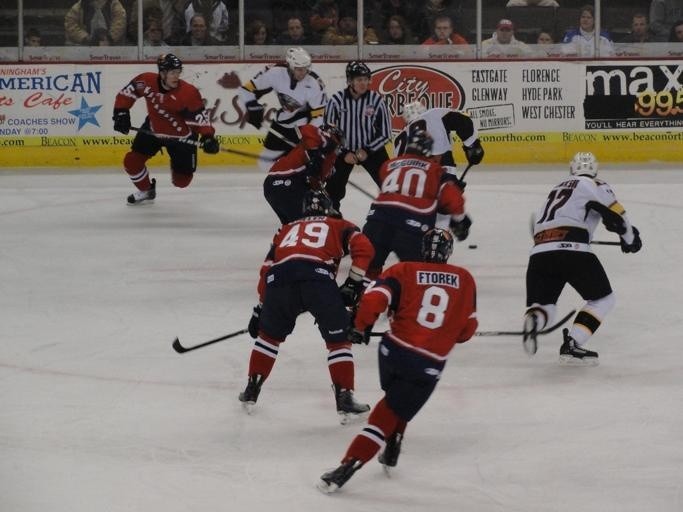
top-left (401, 101), bottom-right (428, 125)
top-left (157, 53), bottom-right (183, 71)
top-left (569, 152), bottom-right (599, 177)
top-left (317, 123), bottom-right (344, 147)
top-left (285, 46), bottom-right (312, 71)
top-left (301, 188), bottom-right (333, 215)
top-left (406, 129), bottom-right (435, 158)
top-left (420, 228), bottom-right (454, 262)
top-left (346, 60), bottom-right (371, 82)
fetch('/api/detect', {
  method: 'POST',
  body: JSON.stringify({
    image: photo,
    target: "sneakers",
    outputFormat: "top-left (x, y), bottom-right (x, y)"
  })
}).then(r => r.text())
top-left (561, 326), bottom-right (600, 359)
top-left (319, 464), bottom-right (356, 489)
top-left (522, 314), bottom-right (539, 356)
top-left (377, 444), bottom-right (401, 467)
top-left (127, 178), bottom-right (157, 204)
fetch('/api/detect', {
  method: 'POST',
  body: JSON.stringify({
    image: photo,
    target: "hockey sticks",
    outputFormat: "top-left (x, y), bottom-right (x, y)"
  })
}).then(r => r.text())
top-left (129, 126), bottom-right (285, 162)
top-left (370, 310), bottom-right (576, 337)
top-left (173, 328), bottom-right (249, 354)
top-left (530, 212), bottom-right (622, 246)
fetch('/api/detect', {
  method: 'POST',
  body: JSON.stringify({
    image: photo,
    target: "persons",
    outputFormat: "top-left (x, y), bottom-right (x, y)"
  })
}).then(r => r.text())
top-left (393, 101), bottom-right (484, 232)
top-left (361, 128), bottom-right (471, 294)
top-left (321, 227), bottom-right (478, 489)
top-left (113, 54), bottom-right (219, 204)
top-left (522, 151), bottom-right (643, 358)
top-left (238, 186), bottom-right (372, 413)
top-left (237, 47), bottom-right (328, 175)
top-left (263, 121), bottom-right (346, 225)
top-left (24, 28), bottom-right (40, 46)
top-left (324, 60), bottom-right (391, 211)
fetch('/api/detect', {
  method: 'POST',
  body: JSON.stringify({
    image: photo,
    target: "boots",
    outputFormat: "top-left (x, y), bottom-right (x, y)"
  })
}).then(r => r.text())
top-left (238, 380), bottom-right (261, 403)
top-left (335, 389), bottom-right (371, 414)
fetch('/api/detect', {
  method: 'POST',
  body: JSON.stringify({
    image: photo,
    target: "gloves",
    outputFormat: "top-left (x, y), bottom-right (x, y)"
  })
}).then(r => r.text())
top-left (346, 323), bottom-right (372, 346)
top-left (338, 277), bottom-right (364, 308)
top-left (112, 110), bottom-right (131, 136)
top-left (243, 100), bottom-right (265, 130)
top-left (198, 134), bottom-right (220, 154)
top-left (248, 304), bottom-right (262, 339)
top-left (305, 147), bottom-right (323, 179)
top-left (448, 214), bottom-right (472, 242)
top-left (619, 225), bottom-right (642, 254)
top-left (461, 138), bottom-right (485, 165)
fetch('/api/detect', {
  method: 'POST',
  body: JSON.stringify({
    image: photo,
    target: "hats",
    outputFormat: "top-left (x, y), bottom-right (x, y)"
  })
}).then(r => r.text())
top-left (496, 18), bottom-right (514, 30)
